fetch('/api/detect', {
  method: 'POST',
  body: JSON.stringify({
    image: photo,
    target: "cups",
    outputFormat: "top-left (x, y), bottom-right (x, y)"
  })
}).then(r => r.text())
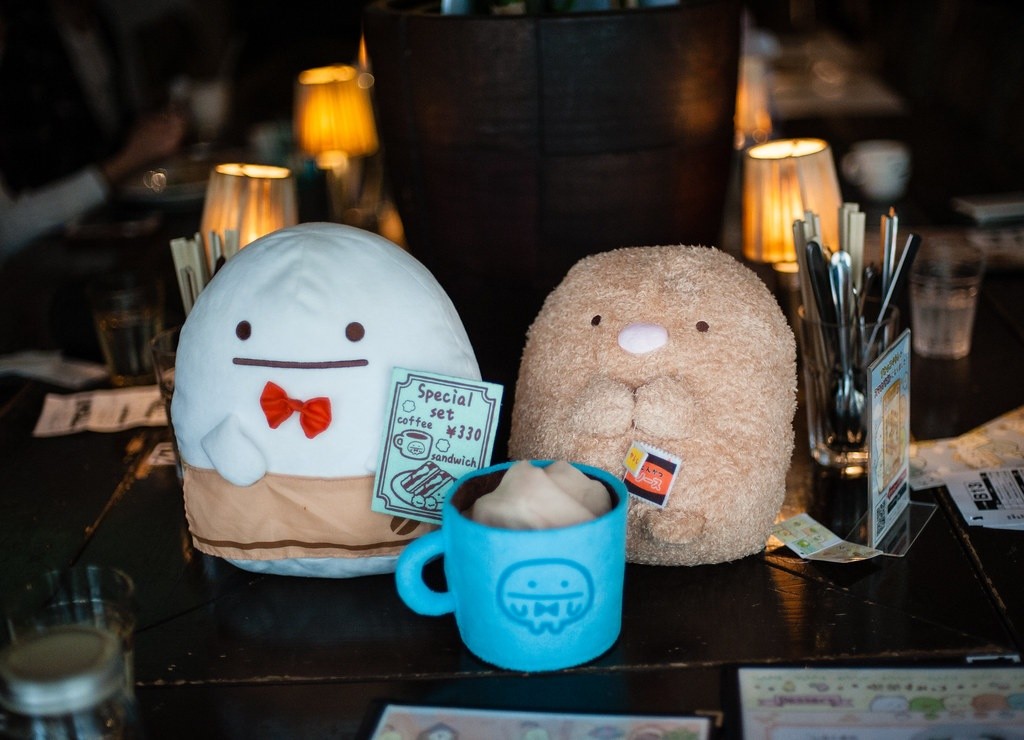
top-left (99, 309), bottom-right (163, 385)
top-left (0, 625), bottom-right (126, 740)
top-left (7, 562), bottom-right (138, 733)
top-left (799, 304), bottom-right (898, 472)
top-left (394, 458), bottom-right (629, 669)
top-left (153, 327), bottom-right (185, 483)
top-left (910, 237), bottom-right (986, 356)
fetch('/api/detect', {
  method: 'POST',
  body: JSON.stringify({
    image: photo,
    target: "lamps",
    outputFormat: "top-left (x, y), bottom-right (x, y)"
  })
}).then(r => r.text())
top-left (294, 65), bottom-right (383, 224)
top-left (200, 162), bottom-right (299, 259)
top-left (743, 138), bottom-right (842, 302)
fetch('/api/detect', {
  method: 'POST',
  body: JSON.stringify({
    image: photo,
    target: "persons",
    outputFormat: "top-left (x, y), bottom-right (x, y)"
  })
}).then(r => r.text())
top-left (1, 0), bottom-right (189, 271)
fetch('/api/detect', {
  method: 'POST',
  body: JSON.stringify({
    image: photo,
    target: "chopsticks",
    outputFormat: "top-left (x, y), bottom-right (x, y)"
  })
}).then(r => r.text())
top-left (793, 203), bottom-right (867, 401)
top-left (172, 228), bottom-right (240, 320)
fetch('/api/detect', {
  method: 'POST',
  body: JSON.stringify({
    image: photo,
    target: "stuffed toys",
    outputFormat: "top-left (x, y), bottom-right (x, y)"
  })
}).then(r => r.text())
top-left (168, 220), bottom-right (490, 581)
top-left (506, 241), bottom-right (802, 571)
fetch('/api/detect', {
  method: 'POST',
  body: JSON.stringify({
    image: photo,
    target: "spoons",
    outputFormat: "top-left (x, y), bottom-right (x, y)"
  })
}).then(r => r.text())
top-left (808, 208), bottom-right (917, 452)
